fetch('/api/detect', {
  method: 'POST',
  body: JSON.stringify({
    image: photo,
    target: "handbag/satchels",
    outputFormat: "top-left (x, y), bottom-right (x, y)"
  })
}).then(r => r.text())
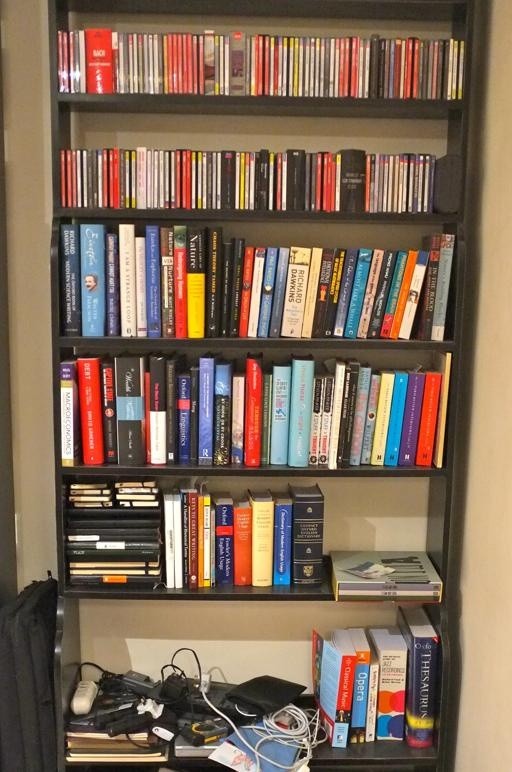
top-left (0, 579), bottom-right (58, 772)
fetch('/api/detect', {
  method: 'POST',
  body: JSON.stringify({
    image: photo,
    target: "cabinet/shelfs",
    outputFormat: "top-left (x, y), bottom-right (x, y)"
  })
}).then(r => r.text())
top-left (47, 0), bottom-right (485, 771)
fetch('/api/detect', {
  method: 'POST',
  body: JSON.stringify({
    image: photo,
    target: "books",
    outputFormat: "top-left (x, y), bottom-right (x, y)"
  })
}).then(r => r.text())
top-left (57, 29), bottom-right (468, 102)
top-left (60, 349), bottom-right (453, 469)
top-left (60, 223), bottom-right (456, 342)
top-left (59, 149), bottom-right (438, 215)
top-left (65, 476), bottom-right (444, 602)
top-left (65, 709), bottom-right (169, 765)
top-left (310, 601), bottom-right (441, 752)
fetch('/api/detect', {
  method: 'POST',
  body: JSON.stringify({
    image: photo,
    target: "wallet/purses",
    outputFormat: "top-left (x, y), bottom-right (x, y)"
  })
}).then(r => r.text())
top-left (225, 675), bottom-right (308, 717)
